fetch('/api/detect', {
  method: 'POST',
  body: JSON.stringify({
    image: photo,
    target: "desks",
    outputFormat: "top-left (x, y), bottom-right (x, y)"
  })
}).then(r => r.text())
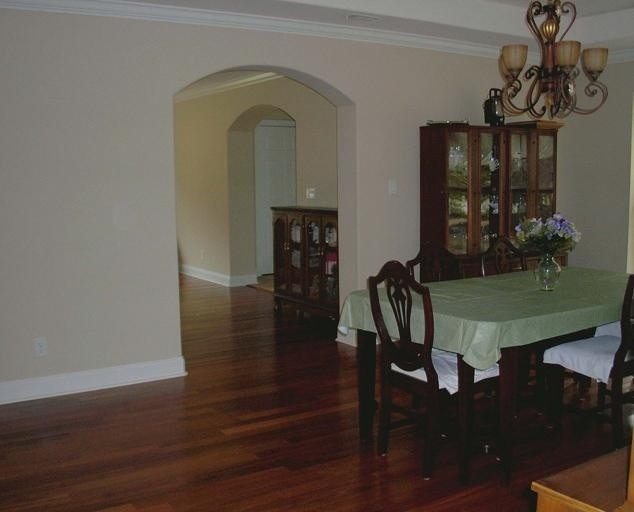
top-left (337, 268), bottom-right (634, 485)
top-left (530, 429), bottom-right (634, 512)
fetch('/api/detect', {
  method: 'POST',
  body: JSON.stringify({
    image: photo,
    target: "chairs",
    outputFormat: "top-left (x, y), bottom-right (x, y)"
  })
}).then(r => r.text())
top-left (542, 274), bottom-right (634, 450)
top-left (367, 261), bottom-right (500, 478)
top-left (481, 236), bottom-right (527, 277)
top-left (405, 241), bottom-right (459, 285)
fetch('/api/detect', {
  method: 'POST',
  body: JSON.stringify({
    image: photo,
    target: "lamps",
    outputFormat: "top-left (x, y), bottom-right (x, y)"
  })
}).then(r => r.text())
top-left (498, 0), bottom-right (608, 119)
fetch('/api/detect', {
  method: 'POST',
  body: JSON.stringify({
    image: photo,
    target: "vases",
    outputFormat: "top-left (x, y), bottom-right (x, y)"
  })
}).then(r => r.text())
top-left (535, 255), bottom-right (562, 291)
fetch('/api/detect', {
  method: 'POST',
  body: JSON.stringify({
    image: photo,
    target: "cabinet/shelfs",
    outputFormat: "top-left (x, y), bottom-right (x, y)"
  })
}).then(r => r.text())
top-left (419, 125), bottom-right (557, 281)
top-left (270, 206), bottom-right (338, 320)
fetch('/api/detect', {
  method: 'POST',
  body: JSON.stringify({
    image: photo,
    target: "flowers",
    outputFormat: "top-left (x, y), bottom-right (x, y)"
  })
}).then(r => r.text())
top-left (514, 212), bottom-right (581, 255)
